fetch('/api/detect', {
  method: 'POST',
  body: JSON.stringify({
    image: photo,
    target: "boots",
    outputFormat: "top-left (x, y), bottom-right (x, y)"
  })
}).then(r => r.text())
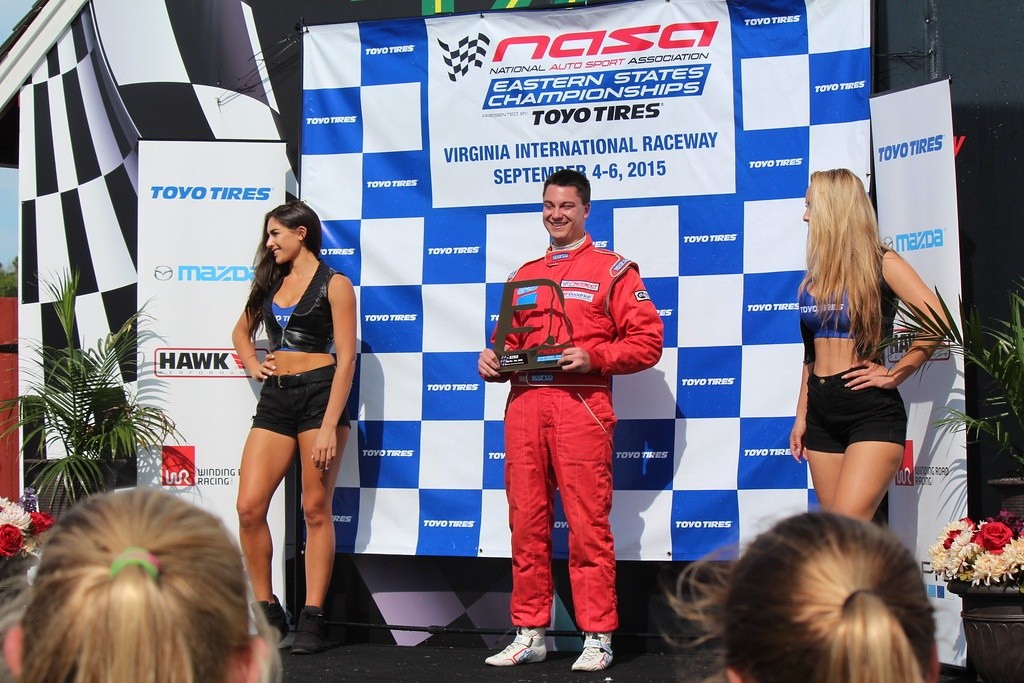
top-left (290, 607), bottom-right (324, 655)
top-left (249, 594), bottom-right (293, 653)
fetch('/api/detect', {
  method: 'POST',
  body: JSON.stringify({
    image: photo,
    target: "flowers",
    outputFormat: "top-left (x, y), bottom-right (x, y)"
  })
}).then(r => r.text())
top-left (0, 487), bottom-right (55, 559)
top-left (928, 509), bottom-right (1024, 592)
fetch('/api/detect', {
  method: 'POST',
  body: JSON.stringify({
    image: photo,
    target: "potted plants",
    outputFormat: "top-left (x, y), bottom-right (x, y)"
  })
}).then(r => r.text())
top-left (868, 275), bottom-right (1024, 520)
top-left (0, 266), bottom-right (190, 522)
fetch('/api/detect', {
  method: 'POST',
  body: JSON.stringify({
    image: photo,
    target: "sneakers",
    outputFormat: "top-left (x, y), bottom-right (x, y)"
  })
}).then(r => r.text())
top-left (572, 632), bottom-right (613, 671)
top-left (484, 626), bottom-right (547, 666)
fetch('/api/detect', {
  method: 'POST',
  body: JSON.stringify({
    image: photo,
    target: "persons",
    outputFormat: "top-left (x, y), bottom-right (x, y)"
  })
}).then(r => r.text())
top-left (477, 170), bottom-right (664, 671)
top-left (0, 487), bottom-right (281, 683)
top-left (789, 169), bottom-right (952, 521)
top-left (722, 511), bottom-right (939, 683)
top-left (232, 201), bottom-right (357, 654)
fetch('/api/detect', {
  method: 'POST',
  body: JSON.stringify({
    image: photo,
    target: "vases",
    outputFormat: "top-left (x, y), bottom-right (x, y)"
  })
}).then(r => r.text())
top-left (0, 554), bottom-right (39, 591)
top-left (947, 580), bottom-right (1024, 683)
